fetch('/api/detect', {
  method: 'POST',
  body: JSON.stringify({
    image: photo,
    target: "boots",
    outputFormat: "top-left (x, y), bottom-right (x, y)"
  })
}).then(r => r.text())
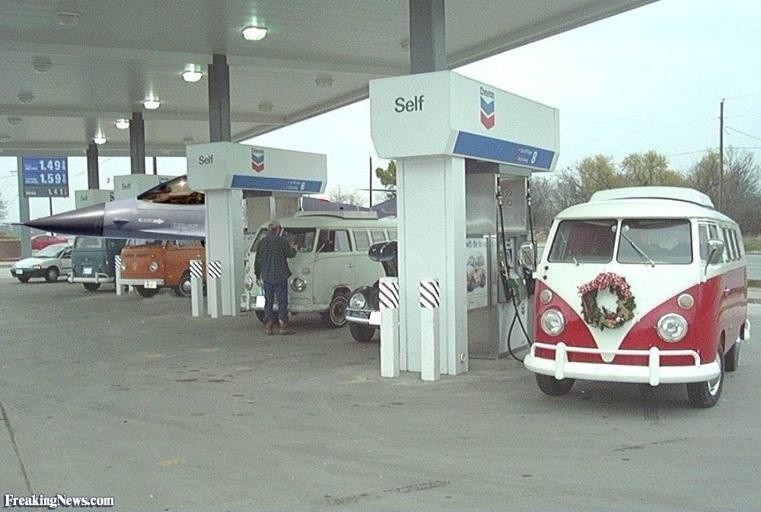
top-left (279, 324), bottom-right (296, 334)
top-left (264, 320), bottom-right (273, 334)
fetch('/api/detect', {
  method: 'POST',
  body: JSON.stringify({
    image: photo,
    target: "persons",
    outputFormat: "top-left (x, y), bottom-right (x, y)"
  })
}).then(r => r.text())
top-left (254, 221), bottom-right (298, 336)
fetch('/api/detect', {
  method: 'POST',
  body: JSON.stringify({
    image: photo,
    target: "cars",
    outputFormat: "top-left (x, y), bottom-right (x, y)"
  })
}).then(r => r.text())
top-left (343, 240), bottom-right (397, 342)
top-left (10, 242), bottom-right (73, 282)
top-left (31, 234), bottom-right (65, 250)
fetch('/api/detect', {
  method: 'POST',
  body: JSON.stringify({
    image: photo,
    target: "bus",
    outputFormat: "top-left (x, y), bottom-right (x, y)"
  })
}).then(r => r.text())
top-left (239, 208), bottom-right (398, 329)
top-left (522, 185), bottom-right (748, 408)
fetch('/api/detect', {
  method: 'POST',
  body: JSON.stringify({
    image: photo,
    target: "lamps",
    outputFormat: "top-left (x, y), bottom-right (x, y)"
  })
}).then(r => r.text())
top-left (56, 12), bottom-right (80, 26)
top-left (239, 24), bottom-right (266, 41)
top-left (181, 64), bottom-right (205, 82)
top-left (115, 119), bottom-right (132, 130)
top-left (19, 94), bottom-right (35, 104)
top-left (141, 98), bottom-right (164, 111)
top-left (7, 116), bottom-right (21, 127)
top-left (2, 137), bottom-right (11, 143)
top-left (30, 61), bottom-right (55, 72)
top-left (93, 135), bottom-right (107, 145)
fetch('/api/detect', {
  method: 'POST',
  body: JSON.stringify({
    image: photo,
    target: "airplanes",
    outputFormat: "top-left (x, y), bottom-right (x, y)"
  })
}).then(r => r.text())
top-left (8, 175), bottom-right (398, 241)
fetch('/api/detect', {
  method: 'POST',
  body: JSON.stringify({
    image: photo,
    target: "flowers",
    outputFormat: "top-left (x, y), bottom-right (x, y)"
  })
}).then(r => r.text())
top-left (577, 272), bottom-right (636, 331)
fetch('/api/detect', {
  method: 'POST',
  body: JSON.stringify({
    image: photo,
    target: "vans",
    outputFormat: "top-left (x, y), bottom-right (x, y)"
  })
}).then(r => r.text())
top-left (119, 239), bottom-right (206, 297)
top-left (68, 235), bottom-right (126, 291)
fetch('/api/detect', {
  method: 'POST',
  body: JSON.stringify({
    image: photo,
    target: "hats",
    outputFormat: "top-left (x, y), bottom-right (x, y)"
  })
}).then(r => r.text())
top-left (268, 220), bottom-right (282, 230)
